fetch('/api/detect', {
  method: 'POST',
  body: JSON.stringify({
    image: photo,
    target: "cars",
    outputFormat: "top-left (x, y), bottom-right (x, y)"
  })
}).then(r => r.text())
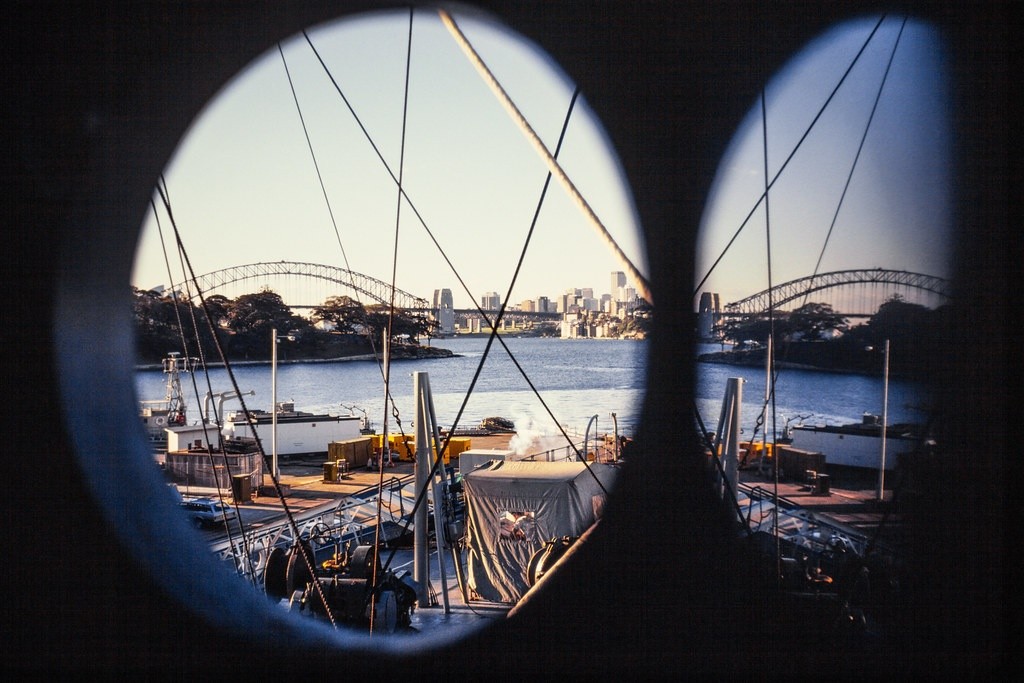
top-left (180, 498), bottom-right (237, 530)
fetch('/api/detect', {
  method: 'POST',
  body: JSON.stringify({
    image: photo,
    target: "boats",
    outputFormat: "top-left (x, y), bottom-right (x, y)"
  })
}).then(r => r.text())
top-left (164, 392), bottom-right (375, 463)
top-left (136, 352), bottom-right (200, 454)
top-left (793, 412), bottom-right (911, 437)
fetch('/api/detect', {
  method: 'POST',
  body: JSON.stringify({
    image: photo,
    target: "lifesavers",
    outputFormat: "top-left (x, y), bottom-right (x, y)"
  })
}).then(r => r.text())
top-left (155, 416), bottom-right (165, 425)
